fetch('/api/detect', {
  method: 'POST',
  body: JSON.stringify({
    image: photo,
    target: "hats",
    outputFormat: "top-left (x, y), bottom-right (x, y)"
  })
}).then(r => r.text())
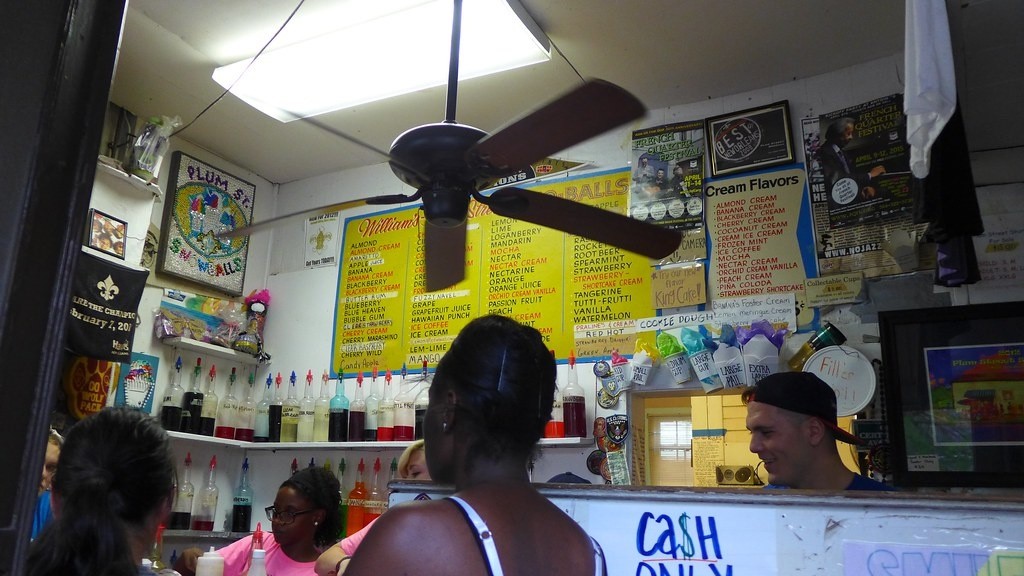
top-left (747, 372), bottom-right (866, 445)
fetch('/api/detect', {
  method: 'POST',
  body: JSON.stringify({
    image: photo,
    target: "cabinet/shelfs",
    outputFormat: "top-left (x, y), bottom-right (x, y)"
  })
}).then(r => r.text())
top-left (166, 432), bottom-right (593, 541)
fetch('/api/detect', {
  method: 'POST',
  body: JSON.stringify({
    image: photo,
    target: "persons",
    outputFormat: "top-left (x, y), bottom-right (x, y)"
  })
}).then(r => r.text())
top-left (22, 406), bottom-right (176, 576)
top-left (631, 156), bottom-right (691, 199)
top-left (313, 439), bottom-right (431, 576)
top-left (821, 117), bottom-right (893, 213)
top-left (741, 371), bottom-right (897, 492)
top-left (342, 314), bottom-right (608, 576)
top-left (172, 466), bottom-right (341, 576)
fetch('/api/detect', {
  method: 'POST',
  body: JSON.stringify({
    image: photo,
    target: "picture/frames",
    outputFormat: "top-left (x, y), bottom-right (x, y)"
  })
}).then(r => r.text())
top-left (876, 300), bottom-right (1024, 488)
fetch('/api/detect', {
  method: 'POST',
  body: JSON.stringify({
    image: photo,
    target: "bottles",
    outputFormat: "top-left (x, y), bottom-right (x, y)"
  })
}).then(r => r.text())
top-left (232, 457), bottom-right (253, 532)
top-left (246, 522), bottom-right (264, 576)
top-left (168, 452), bottom-right (195, 530)
top-left (160, 355), bottom-right (432, 443)
top-left (245, 549), bottom-right (267, 576)
top-left (195, 546), bottom-right (225, 576)
top-left (543, 349), bottom-right (587, 438)
top-left (138, 525), bottom-right (178, 572)
top-left (192, 454), bottom-right (219, 531)
top-left (788, 320), bottom-right (847, 372)
top-left (289, 456), bottom-right (398, 540)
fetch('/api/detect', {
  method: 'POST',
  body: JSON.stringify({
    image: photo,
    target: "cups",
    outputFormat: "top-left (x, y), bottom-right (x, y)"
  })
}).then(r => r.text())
top-left (612, 348), bottom-right (723, 394)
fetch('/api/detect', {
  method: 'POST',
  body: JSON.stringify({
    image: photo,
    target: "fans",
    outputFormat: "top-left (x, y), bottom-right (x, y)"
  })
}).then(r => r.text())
top-left (216, 0), bottom-right (682, 292)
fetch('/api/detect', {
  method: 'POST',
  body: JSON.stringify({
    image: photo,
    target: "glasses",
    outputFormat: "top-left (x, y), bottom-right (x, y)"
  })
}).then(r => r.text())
top-left (265, 506), bottom-right (319, 524)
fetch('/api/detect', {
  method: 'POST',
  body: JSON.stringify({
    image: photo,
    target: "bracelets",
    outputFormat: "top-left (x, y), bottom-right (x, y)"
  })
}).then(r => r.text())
top-left (336, 556), bottom-right (351, 572)
